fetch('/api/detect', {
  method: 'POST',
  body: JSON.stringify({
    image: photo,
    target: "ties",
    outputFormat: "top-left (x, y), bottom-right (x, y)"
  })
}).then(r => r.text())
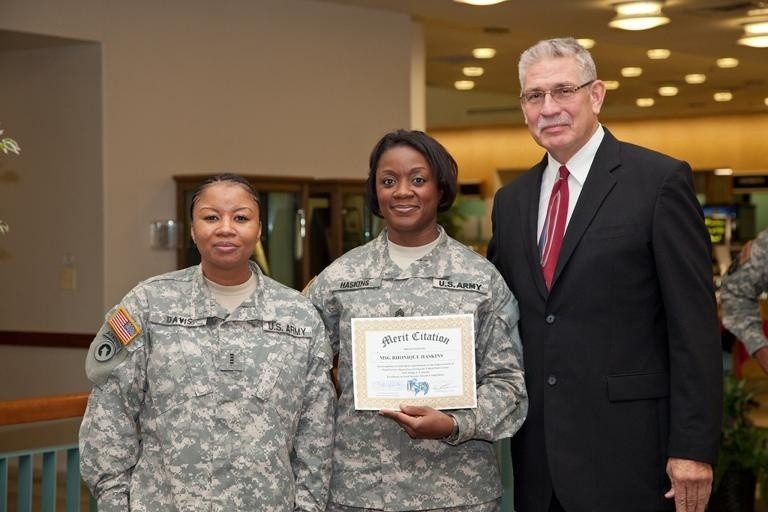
top-left (537, 165), bottom-right (570, 293)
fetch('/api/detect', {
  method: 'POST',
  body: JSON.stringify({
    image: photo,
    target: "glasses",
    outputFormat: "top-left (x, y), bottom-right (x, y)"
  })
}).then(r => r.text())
top-left (519, 80), bottom-right (593, 103)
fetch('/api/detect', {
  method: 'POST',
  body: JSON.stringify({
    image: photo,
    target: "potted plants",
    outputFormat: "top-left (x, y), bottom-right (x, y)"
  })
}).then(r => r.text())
top-left (707, 372), bottom-right (766, 511)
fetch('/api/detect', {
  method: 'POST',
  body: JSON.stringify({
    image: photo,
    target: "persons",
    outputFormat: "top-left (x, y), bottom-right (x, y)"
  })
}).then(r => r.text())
top-left (486, 36), bottom-right (723, 511)
top-left (719, 228), bottom-right (767, 373)
top-left (301, 130), bottom-right (528, 510)
top-left (79, 173), bottom-right (336, 510)
